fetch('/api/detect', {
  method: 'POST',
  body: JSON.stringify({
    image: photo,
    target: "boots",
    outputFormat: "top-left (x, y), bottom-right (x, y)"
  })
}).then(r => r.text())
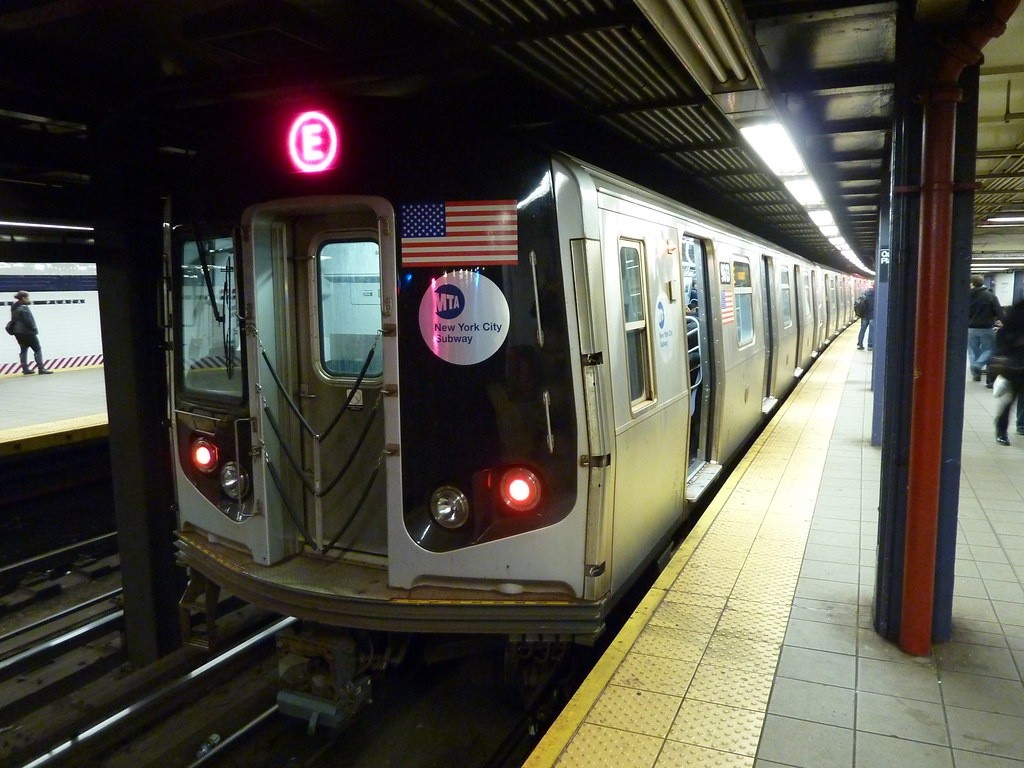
top-left (35, 364), bottom-right (53, 375)
top-left (22, 365), bottom-right (34, 374)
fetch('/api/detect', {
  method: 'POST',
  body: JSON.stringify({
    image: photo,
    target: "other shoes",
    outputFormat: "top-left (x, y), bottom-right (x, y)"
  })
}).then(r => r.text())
top-left (868, 346), bottom-right (873, 351)
top-left (857, 344), bottom-right (864, 350)
top-left (981, 369), bottom-right (987, 375)
top-left (973, 377), bottom-right (981, 382)
top-left (985, 382), bottom-right (993, 389)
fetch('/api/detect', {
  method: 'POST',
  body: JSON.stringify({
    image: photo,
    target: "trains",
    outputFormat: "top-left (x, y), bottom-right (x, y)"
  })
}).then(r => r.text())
top-left (143, 90), bottom-right (876, 727)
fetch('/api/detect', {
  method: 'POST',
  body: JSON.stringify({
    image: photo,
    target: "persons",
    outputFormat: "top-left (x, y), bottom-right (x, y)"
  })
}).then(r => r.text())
top-left (5, 291), bottom-right (54, 375)
top-left (857, 285), bottom-right (873, 351)
top-left (968, 275), bottom-right (1024, 446)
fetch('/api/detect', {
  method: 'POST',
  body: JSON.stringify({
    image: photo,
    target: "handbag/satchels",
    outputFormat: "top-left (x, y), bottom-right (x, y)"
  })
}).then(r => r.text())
top-left (992, 374), bottom-right (1011, 398)
top-left (5, 321), bottom-right (14, 335)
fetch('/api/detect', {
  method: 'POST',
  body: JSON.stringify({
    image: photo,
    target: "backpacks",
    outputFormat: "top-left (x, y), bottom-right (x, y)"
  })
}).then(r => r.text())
top-left (854, 291), bottom-right (868, 318)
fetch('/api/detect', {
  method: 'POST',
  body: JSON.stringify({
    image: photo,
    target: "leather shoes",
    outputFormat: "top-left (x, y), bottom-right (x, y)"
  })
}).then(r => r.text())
top-left (996, 437), bottom-right (1010, 446)
top-left (1017, 430), bottom-right (1024, 436)
